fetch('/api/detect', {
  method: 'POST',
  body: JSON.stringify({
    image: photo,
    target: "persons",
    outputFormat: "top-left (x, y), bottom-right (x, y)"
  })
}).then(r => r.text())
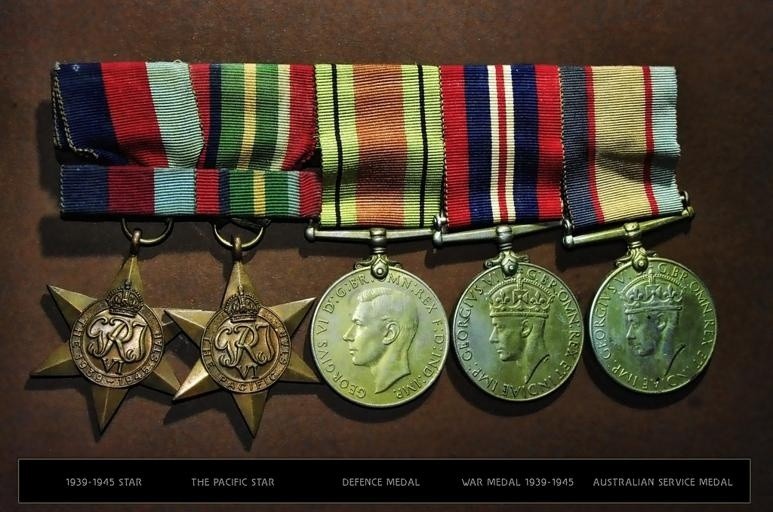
top-left (343, 288), bottom-right (419, 393)
top-left (486, 274), bottom-right (555, 384)
top-left (622, 271), bottom-right (686, 376)
